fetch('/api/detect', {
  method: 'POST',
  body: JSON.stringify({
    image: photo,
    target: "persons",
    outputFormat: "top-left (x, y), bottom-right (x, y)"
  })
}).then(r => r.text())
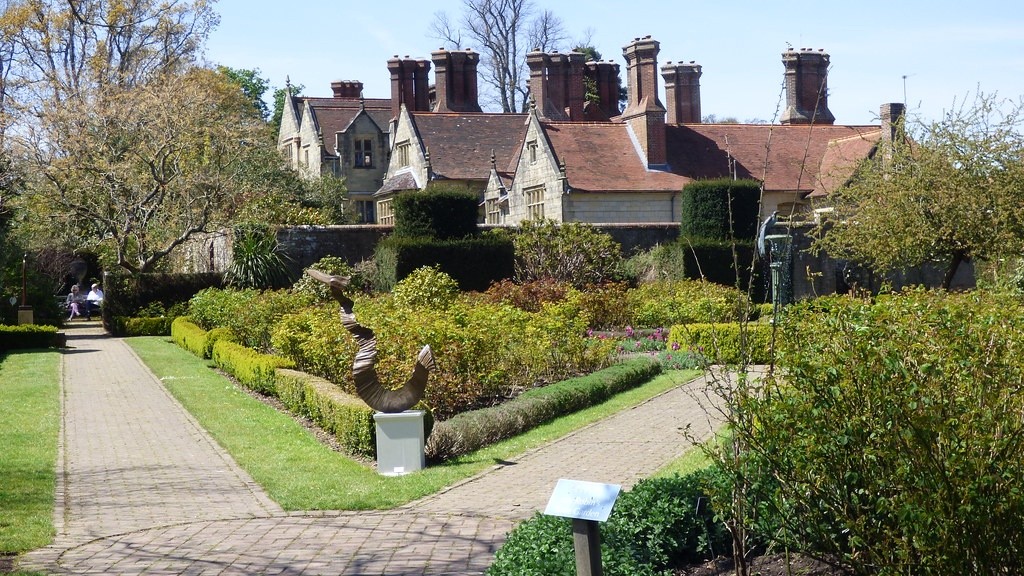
top-left (85, 284), bottom-right (103, 321)
top-left (66, 285), bottom-right (83, 322)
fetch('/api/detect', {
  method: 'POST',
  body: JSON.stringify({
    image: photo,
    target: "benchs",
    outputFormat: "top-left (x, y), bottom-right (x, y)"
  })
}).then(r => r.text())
top-left (61, 299), bottom-right (103, 319)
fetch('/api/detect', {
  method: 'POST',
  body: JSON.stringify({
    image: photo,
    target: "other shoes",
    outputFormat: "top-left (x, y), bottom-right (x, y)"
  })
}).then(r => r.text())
top-left (86, 318), bottom-right (91, 321)
top-left (87, 312), bottom-right (90, 317)
top-left (76, 313), bottom-right (80, 316)
top-left (67, 318), bottom-right (72, 321)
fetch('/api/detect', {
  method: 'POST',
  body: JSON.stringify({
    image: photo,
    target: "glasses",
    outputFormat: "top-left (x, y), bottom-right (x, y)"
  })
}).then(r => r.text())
top-left (73, 290), bottom-right (77, 291)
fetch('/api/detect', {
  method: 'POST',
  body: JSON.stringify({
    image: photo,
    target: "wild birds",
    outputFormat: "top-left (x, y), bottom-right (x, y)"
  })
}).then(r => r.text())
top-left (759, 211), bottom-right (780, 256)
